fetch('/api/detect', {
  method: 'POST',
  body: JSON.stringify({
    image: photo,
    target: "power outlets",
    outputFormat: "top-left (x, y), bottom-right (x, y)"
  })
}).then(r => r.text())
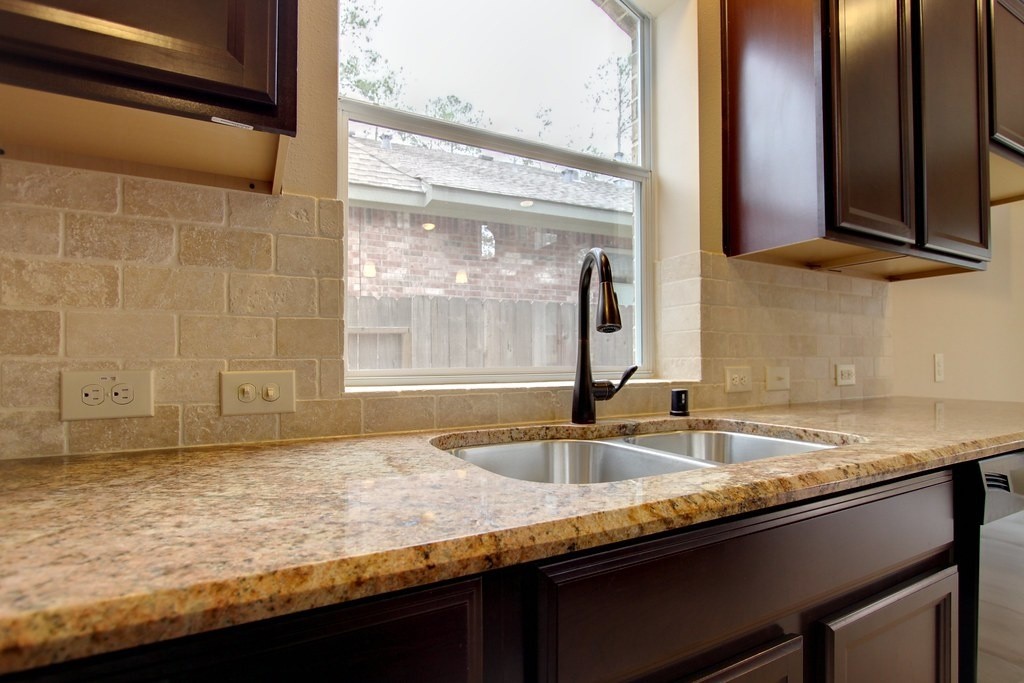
top-left (56, 366), bottom-right (157, 425)
top-left (932, 402), bottom-right (947, 431)
top-left (723, 365), bottom-right (755, 393)
top-left (832, 363), bottom-right (856, 387)
top-left (835, 412), bottom-right (860, 430)
top-left (933, 351), bottom-right (947, 384)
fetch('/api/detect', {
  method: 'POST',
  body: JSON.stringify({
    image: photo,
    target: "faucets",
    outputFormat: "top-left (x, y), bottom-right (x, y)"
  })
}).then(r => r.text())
top-left (571, 247), bottom-right (638, 425)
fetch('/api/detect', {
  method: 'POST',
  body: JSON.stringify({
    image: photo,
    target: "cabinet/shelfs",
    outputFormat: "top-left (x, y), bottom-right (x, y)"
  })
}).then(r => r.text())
top-left (0, 573), bottom-right (488, 683)
top-left (0, 0), bottom-right (299, 196)
top-left (718, 0), bottom-right (993, 285)
top-left (988, 0), bottom-right (1024, 209)
top-left (524, 471), bottom-right (961, 683)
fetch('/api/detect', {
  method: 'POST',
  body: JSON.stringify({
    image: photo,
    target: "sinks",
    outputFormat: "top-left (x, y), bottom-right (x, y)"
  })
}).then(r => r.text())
top-left (623, 430), bottom-right (837, 467)
top-left (440, 438), bottom-right (716, 485)
